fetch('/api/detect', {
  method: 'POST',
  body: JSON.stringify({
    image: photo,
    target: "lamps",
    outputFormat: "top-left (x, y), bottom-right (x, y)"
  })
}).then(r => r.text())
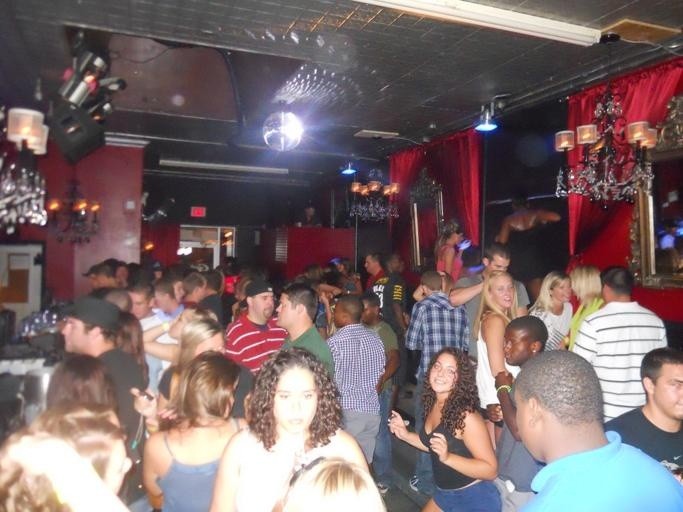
top-left (554, 31), bottom-right (658, 212)
top-left (473, 94), bottom-right (511, 133)
top-left (260, 99), bottom-right (303, 153)
top-left (47, 177), bottom-right (101, 245)
top-left (348, 168), bottom-right (400, 225)
top-left (0, 104), bottom-right (51, 236)
top-left (338, 160), bottom-right (359, 176)
top-left (50, 29), bottom-right (128, 166)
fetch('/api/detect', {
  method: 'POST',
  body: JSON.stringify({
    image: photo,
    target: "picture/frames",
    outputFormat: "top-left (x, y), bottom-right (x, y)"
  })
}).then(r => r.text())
top-left (407, 166), bottom-right (445, 268)
top-left (626, 93), bottom-right (683, 291)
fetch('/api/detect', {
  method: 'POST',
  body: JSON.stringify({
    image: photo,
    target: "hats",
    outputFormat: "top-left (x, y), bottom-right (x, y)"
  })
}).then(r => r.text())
top-left (238, 281), bottom-right (273, 308)
top-left (59, 298), bottom-right (121, 334)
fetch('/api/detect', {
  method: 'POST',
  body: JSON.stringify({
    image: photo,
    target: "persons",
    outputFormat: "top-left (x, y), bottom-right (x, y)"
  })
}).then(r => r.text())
top-left (0, 192), bottom-right (683, 512)
